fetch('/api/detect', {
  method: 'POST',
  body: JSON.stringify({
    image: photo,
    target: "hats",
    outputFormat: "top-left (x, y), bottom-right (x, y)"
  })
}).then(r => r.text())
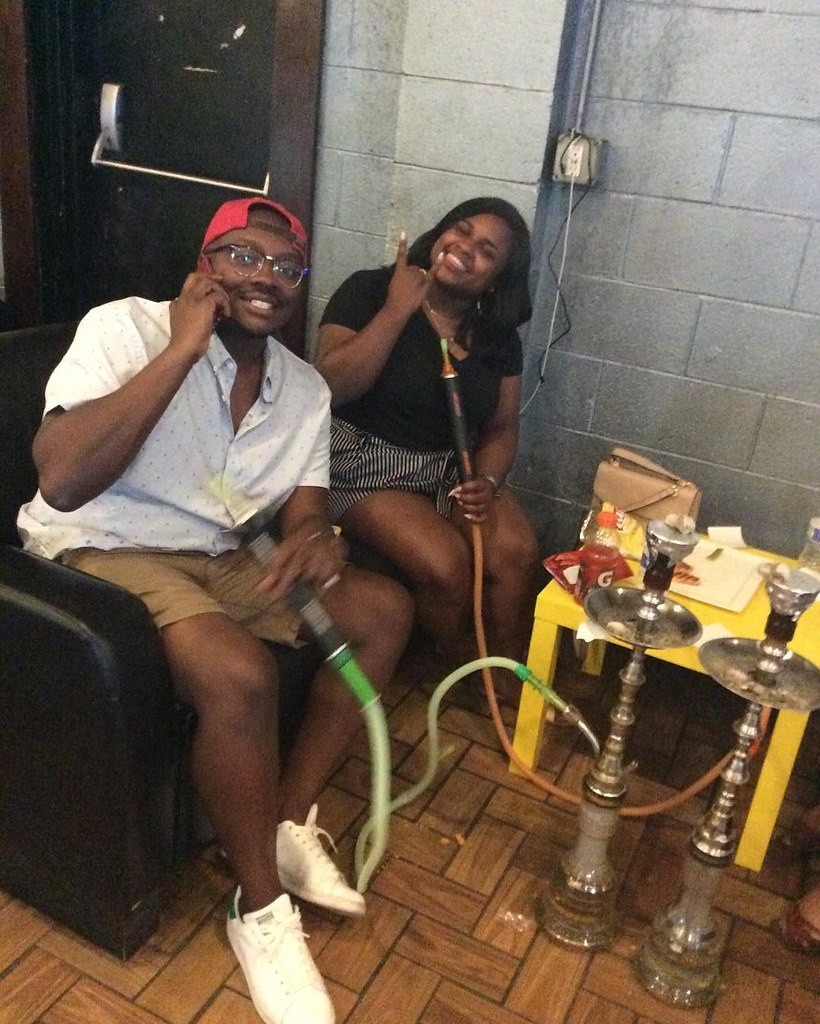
top-left (200, 196), bottom-right (308, 267)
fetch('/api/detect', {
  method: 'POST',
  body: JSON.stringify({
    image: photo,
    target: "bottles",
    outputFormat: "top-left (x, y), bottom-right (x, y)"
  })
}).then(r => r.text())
top-left (639, 542), bottom-right (657, 584)
top-left (574, 506), bottom-right (624, 605)
top-left (796, 516), bottom-right (820, 579)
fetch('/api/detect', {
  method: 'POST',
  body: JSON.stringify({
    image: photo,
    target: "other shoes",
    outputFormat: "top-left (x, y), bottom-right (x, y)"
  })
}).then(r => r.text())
top-left (780, 888), bottom-right (820, 954)
top-left (778, 805), bottom-right (820, 863)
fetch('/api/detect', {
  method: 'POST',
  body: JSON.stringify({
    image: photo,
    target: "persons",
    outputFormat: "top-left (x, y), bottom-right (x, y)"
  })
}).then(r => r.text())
top-left (316, 197), bottom-right (584, 727)
top-left (16, 197), bottom-right (413, 1024)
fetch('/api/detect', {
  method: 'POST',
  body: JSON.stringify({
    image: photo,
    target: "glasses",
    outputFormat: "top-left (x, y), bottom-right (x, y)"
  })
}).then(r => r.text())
top-left (204, 243), bottom-right (312, 290)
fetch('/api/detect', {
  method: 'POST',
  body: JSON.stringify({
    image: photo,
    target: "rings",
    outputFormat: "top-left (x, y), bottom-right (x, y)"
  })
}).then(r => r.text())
top-left (418, 267), bottom-right (429, 277)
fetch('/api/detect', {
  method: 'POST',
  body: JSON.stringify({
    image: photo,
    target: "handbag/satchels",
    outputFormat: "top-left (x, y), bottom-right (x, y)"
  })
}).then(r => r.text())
top-left (590, 446), bottom-right (703, 536)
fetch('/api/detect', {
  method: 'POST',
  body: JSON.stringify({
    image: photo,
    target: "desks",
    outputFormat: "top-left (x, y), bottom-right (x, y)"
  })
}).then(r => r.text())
top-left (508, 530), bottom-right (820, 873)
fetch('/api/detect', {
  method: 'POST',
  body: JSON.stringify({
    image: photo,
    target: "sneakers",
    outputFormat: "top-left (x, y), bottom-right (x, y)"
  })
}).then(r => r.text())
top-left (225, 885), bottom-right (336, 1024)
top-left (275, 803), bottom-right (368, 919)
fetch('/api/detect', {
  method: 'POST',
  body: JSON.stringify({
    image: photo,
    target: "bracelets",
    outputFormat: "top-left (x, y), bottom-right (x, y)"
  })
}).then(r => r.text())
top-left (484, 473), bottom-right (502, 498)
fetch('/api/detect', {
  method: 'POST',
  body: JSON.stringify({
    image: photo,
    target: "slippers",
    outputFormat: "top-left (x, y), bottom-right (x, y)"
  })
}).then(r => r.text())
top-left (420, 668), bottom-right (505, 719)
top-left (478, 672), bottom-right (571, 726)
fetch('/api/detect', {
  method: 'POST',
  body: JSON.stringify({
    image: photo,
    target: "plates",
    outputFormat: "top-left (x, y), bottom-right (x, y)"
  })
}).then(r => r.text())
top-left (672, 538), bottom-right (773, 612)
top-left (582, 586), bottom-right (703, 648)
top-left (698, 638), bottom-right (820, 710)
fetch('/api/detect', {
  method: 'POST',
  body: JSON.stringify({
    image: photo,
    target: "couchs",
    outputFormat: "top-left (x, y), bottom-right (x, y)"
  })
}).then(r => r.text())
top-left (1, 318), bottom-right (322, 960)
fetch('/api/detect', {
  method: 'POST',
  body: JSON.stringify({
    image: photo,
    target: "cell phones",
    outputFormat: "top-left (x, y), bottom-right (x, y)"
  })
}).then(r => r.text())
top-left (199, 258), bottom-right (223, 329)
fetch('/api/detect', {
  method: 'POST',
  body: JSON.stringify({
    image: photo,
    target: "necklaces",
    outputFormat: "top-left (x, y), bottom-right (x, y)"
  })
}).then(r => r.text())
top-left (423, 297), bottom-right (457, 345)
